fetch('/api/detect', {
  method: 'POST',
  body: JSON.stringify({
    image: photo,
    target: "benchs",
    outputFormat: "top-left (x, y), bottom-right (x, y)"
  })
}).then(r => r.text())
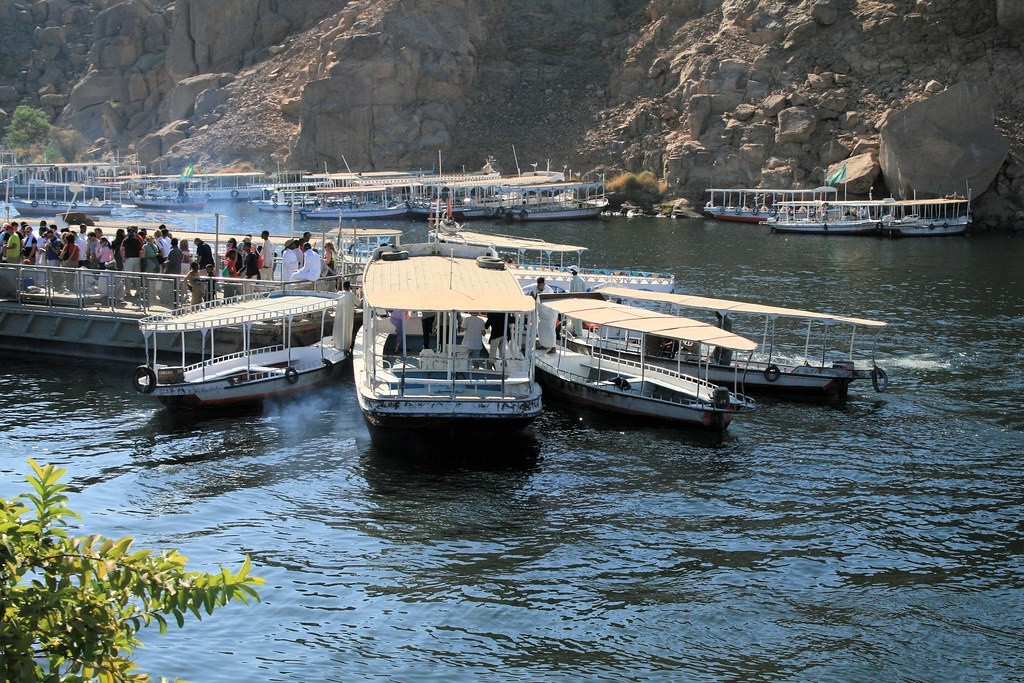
top-left (587, 376), bottom-right (647, 386)
top-left (249, 365), bottom-right (299, 375)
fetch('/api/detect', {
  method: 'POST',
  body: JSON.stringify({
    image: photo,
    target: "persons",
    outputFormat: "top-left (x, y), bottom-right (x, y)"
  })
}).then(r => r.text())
top-left (420, 311), bottom-right (436, 350)
top-left (459, 311), bottom-right (486, 367)
top-left (504, 254), bottom-right (514, 263)
top-left (568, 265), bottom-right (587, 291)
top-left (0, 220), bottom-right (337, 310)
top-left (333, 280), bottom-right (361, 349)
top-left (532, 278), bottom-right (558, 354)
top-left (390, 309), bottom-right (404, 353)
top-left (485, 312), bottom-right (515, 367)
top-left (521, 200), bottom-right (531, 209)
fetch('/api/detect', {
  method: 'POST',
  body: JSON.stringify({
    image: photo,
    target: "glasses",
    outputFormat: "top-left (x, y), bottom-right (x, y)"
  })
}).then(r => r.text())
top-left (242, 239), bottom-right (248, 243)
top-left (227, 240), bottom-right (233, 243)
top-left (47, 235), bottom-right (53, 238)
top-left (24, 230), bottom-right (30, 233)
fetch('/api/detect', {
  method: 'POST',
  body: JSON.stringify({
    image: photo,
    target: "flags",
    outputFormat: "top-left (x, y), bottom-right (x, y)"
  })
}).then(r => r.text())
top-left (179, 162), bottom-right (194, 181)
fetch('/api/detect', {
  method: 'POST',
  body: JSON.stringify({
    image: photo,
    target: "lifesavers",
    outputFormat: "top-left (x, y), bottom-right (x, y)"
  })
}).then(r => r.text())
top-left (422, 200), bottom-right (431, 208)
top-left (141, 196), bottom-right (170, 201)
top-left (506, 212), bottom-right (513, 220)
top-left (930, 223), bottom-right (948, 230)
top-left (412, 199), bottom-right (419, 207)
top-left (322, 358), bottom-right (333, 376)
top-left (301, 214), bottom-right (307, 221)
top-left (440, 220), bottom-right (460, 232)
top-left (288, 202), bottom-right (292, 206)
top-left (718, 206), bottom-right (816, 217)
top-left (300, 202), bottom-right (305, 207)
top-left (476, 256), bottom-right (505, 270)
top-left (484, 207), bottom-right (494, 217)
top-left (497, 207), bottom-right (505, 215)
top-left (285, 367), bottom-right (299, 384)
top-left (876, 222), bottom-right (883, 231)
top-left (273, 203), bottom-right (277, 208)
top-left (381, 250), bottom-right (409, 260)
top-left (231, 190), bottom-right (239, 197)
top-left (133, 364), bottom-right (156, 394)
top-left (519, 210), bottom-right (529, 219)
top-left (764, 366), bottom-right (780, 382)
top-left (344, 350), bottom-right (351, 359)
top-left (872, 368), bottom-right (888, 393)
top-left (31, 200), bottom-right (77, 209)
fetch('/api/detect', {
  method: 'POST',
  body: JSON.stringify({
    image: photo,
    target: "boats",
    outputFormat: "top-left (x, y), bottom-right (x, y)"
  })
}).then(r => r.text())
top-left (352, 243), bottom-right (545, 446)
top-left (703, 186), bottom-right (838, 222)
top-left (768, 200), bottom-right (891, 231)
top-left (532, 290), bottom-right (758, 436)
top-left (0, 164), bottom-right (611, 222)
top-left (595, 288), bottom-right (889, 399)
top-left (881, 199), bottom-right (971, 234)
top-left (134, 289), bottom-right (351, 409)
top-left (428, 226), bottom-right (591, 269)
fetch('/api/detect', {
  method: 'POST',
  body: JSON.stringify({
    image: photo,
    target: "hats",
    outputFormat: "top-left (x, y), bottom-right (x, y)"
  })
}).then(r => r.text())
top-left (284, 238), bottom-right (294, 247)
top-left (5, 224), bottom-right (13, 230)
top-left (567, 265), bottom-right (580, 273)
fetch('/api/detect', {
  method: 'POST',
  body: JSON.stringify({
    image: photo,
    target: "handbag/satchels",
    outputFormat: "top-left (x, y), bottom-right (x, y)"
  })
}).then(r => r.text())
top-left (105, 259), bottom-right (116, 268)
top-left (60, 260), bottom-right (69, 267)
